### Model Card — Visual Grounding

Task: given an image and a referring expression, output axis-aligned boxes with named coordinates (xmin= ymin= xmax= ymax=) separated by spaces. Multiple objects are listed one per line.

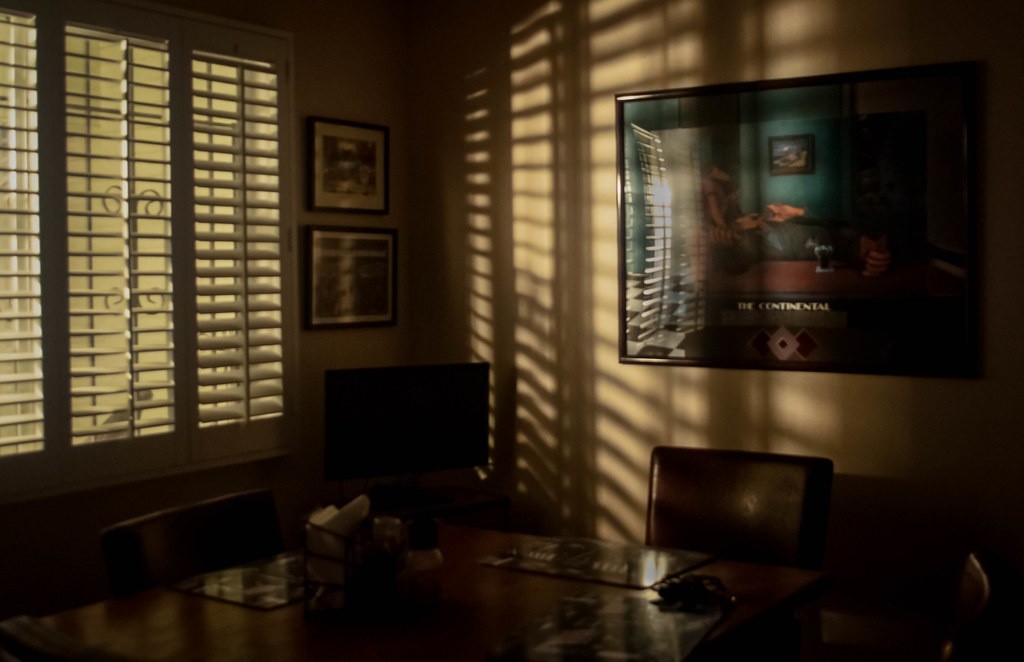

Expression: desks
xmin=0 ymin=524 xmax=836 ymax=662
xmin=372 ymin=487 xmax=514 ymax=531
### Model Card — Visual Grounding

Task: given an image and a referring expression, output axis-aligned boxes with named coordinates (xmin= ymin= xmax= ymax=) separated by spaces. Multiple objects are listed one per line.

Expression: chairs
xmin=98 ymin=486 xmax=286 ymax=596
xmin=941 ymin=544 xmax=1024 ymax=662
xmin=643 ymin=445 xmax=834 ymax=571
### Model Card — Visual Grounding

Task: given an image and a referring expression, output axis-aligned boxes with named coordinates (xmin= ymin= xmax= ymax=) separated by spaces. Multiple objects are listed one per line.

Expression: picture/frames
xmin=302 ymin=115 xmax=390 ymax=216
xmin=614 ymin=61 xmax=986 ymax=379
xmin=301 ymin=223 xmax=399 ymax=331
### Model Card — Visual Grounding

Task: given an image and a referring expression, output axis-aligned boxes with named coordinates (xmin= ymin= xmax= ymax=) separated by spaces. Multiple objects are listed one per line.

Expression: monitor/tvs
xmin=324 ymin=362 xmax=489 ymax=510
